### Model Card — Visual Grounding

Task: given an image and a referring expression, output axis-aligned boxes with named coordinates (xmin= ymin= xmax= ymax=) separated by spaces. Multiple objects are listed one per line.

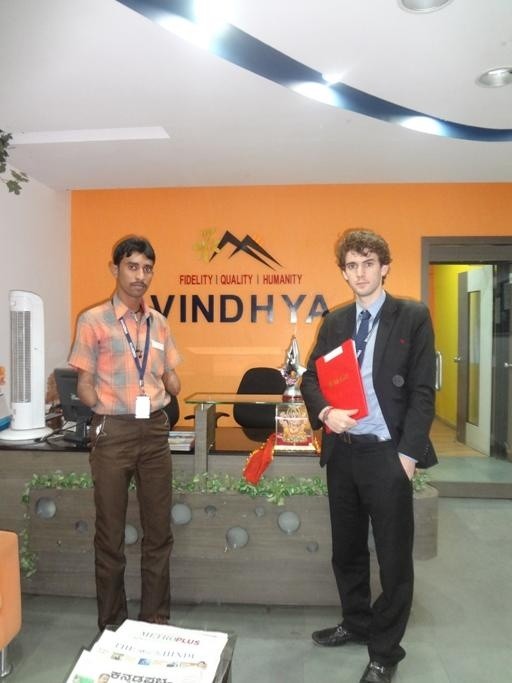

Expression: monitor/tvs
xmin=53 ymin=369 xmax=96 ymax=443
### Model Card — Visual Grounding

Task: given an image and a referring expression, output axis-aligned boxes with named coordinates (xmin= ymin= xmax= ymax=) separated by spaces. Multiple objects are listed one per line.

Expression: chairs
xmin=184 ymin=365 xmax=290 ymax=432
xmin=0 ymin=524 xmax=25 ymax=682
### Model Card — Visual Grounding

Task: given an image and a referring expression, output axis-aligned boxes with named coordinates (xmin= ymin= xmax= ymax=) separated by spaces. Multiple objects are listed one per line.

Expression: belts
xmin=109 ymin=410 xmax=162 ymax=421
xmin=336 ymin=432 xmax=387 ymax=445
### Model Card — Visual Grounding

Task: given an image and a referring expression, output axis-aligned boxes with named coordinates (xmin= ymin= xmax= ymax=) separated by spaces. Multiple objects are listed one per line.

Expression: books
xmin=314 ymin=336 xmax=369 ymax=436
xmin=67 ymin=617 xmax=230 ymax=683
xmin=167 ymin=428 xmax=196 ymax=452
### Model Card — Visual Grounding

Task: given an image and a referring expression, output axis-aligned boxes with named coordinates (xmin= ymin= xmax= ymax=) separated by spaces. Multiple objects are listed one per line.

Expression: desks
xmin=54 ymin=618 xmax=242 ymax=682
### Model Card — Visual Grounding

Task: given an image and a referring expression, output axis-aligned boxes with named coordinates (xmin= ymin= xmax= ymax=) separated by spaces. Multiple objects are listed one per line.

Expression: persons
xmin=67 ymin=232 xmax=183 ymax=632
xmin=298 ymin=225 xmax=436 ymax=682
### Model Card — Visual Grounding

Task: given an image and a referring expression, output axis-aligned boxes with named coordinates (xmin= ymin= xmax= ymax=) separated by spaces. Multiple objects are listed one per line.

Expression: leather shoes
xmin=359 ymin=659 xmax=398 ymax=682
xmin=311 ymin=623 xmax=373 ymax=648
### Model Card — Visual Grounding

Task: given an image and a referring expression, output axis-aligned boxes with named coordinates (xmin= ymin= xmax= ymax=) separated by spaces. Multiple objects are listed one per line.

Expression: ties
xmin=354 ymin=309 xmax=372 ymax=368
